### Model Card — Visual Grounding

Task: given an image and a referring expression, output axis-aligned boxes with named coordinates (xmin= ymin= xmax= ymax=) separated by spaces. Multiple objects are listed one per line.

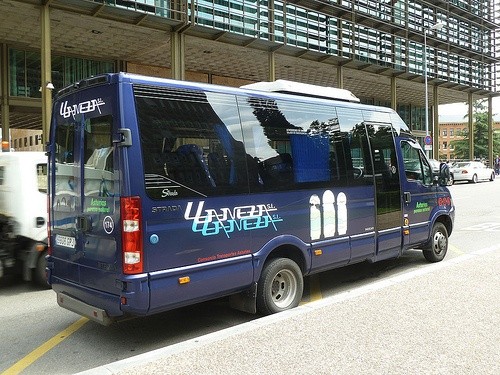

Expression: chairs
xmin=149 ymin=139 xmax=294 ymax=194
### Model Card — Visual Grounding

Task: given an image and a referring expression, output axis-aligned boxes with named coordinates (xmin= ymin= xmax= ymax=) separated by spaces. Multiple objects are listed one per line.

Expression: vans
xmin=0 ymin=142 xmax=54 ymax=289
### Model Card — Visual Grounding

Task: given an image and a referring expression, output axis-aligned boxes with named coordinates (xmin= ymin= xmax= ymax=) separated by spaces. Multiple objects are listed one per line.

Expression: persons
xmin=494 ymin=154 xmax=500 ymax=175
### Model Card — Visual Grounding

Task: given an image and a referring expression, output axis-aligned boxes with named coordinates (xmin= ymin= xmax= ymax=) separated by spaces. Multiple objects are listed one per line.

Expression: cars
xmin=450 ymin=162 xmax=496 ymax=183
xmin=385 ymin=158 xmax=454 ymax=188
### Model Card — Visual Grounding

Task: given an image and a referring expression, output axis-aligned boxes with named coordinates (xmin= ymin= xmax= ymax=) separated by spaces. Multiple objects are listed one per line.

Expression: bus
xmin=43 ymin=71 xmax=456 ymax=326
